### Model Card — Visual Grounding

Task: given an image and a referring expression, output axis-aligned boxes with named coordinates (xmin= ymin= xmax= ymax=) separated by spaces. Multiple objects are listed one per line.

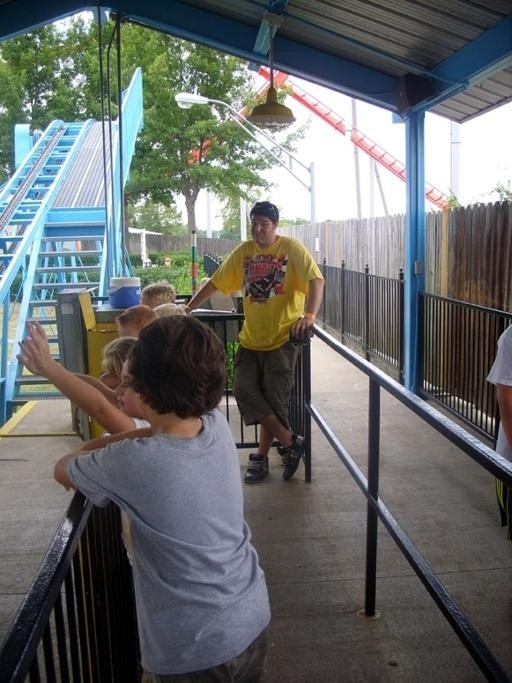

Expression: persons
xmin=52 ymin=315 xmax=275 ymax=682
xmin=183 ymin=199 xmax=325 ymax=487
xmin=483 ymin=321 xmax=512 ymax=538
xmin=28 ymin=279 xmax=180 ymax=567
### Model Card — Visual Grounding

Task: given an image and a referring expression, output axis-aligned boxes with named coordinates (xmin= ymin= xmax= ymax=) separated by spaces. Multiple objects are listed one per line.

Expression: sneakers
xmin=278 ymin=433 xmax=306 ymax=478
xmin=244 ymin=454 xmax=268 ymax=483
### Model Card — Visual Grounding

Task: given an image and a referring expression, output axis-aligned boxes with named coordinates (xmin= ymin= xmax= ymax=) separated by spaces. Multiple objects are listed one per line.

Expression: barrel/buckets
xmin=106 ymin=277 xmax=141 ymax=309
